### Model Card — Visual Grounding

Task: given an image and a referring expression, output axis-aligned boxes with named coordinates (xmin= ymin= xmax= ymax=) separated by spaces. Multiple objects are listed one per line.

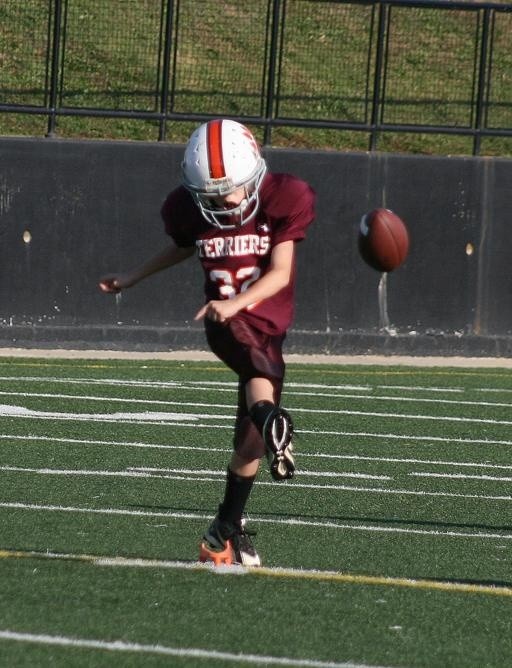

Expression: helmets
xmin=181 ymin=115 xmax=267 ymax=198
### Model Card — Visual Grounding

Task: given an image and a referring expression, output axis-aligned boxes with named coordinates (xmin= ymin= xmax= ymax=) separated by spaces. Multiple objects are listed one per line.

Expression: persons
xmin=98 ymin=116 xmax=315 ymax=569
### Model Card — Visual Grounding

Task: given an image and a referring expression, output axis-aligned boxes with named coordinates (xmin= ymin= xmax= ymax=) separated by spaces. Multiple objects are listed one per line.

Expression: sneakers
xmin=203 ymin=512 xmax=262 ymax=566
xmin=260 ymin=405 xmax=297 ymax=481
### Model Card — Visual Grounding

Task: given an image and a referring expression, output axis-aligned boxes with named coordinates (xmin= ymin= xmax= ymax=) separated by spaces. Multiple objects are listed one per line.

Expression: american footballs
xmin=358 ymin=209 xmax=410 ymax=272
